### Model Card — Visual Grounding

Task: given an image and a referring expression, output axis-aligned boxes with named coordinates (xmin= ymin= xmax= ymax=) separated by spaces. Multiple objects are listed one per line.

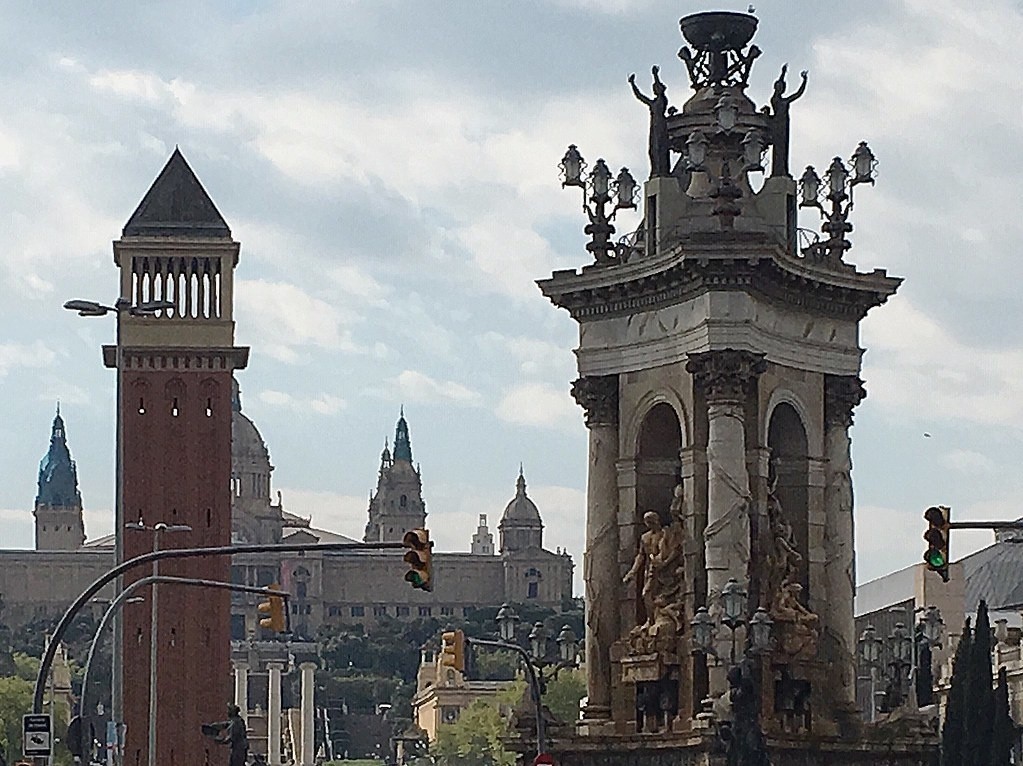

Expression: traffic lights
xmin=921 ymin=505 xmax=951 ymax=575
xmin=443 ymin=628 xmax=464 ymax=671
xmin=257 ymin=585 xmax=287 ymax=633
xmin=402 ymin=529 xmax=434 ymax=593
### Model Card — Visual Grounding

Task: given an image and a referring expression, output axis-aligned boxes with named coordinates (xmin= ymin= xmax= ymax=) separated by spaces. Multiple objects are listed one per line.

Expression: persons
xmin=768 ymin=458 xmax=818 ymax=656
xmin=622 ymin=482 xmax=686 ymax=631
xmin=724 ymin=666 xmax=771 ymax=766
xmin=201 ymin=702 xmax=250 ymax=766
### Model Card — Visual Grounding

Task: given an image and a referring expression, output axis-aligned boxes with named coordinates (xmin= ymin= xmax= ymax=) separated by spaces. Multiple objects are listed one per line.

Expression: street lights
xmin=124 ymin=521 xmax=192 ymax=766
xmin=62 ymin=295 xmax=181 ymax=766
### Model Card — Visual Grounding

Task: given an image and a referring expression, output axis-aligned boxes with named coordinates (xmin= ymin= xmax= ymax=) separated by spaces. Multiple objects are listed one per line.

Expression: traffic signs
xmin=22 ymin=713 xmax=54 ymax=759
